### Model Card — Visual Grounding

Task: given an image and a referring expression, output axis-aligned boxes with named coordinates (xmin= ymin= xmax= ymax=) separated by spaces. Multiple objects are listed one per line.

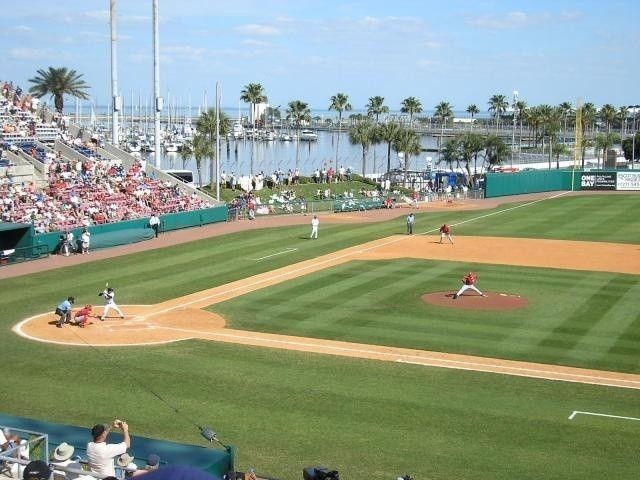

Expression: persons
xmin=50 ymin=230 xmax=78 ymax=256
xmin=439 ymin=224 xmax=455 ymax=246
xmin=0 ymin=427 xmax=161 ymax=480
xmin=150 ymin=213 xmax=161 ymax=239
xmin=406 ymin=213 xmax=415 ymax=236
xmin=84 ymin=419 xmax=132 ymax=479
xmin=0 ymin=78 xmax=214 ymax=236
xmin=309 ymin=214 xmax=320 ymax=240
xmin=217 ymin=165 xmax=470 ymax=221
xmin=72 ymin=302 xmax=94 ymax=329
xmin=79 ymin=227 xmax=91 ymax=255
xmin=54 ymin=296 xmax=76 ymax=328
xmin=97 ymin=286 xmax=127 ymax=322
xmin=453 ymin=271 xmax=488 ymax=300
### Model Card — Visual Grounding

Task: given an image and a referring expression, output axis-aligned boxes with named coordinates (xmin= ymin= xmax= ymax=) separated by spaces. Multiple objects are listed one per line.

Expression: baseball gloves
xmin=462 ymin=280 xmax=465 ymax=283
xmin=93 ymin=314 xmax=98 ymax=318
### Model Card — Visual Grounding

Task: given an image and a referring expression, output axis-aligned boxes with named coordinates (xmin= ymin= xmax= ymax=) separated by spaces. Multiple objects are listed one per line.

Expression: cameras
xmin=117 ymin=422 xmax=123 ymax=426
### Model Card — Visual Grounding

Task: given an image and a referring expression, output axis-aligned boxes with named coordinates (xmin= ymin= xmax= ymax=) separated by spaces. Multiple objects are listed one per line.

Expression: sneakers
xmin=58 ymin=323 xmax=62 ymax=328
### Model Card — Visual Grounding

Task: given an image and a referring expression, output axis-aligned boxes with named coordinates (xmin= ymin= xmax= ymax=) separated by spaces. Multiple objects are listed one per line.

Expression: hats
xmin=146 ymin=454 xmax=160 ymax=466
xmin=23 ymin=460 xmax=53 ymax=479
xmin=54 ymin=442 xmax=74 ymax=461
xmin=92 ymin=423 xmax=108 ymax=436
xmin=69 ymin=297 xmax=74 ymax=304
xmin=118 ymin=453 xmax=134 ymax=466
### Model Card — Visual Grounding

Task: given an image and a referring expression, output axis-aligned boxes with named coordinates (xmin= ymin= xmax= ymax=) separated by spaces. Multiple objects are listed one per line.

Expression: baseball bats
xmin=105 ymin=282 xmax=108 ymax=294
